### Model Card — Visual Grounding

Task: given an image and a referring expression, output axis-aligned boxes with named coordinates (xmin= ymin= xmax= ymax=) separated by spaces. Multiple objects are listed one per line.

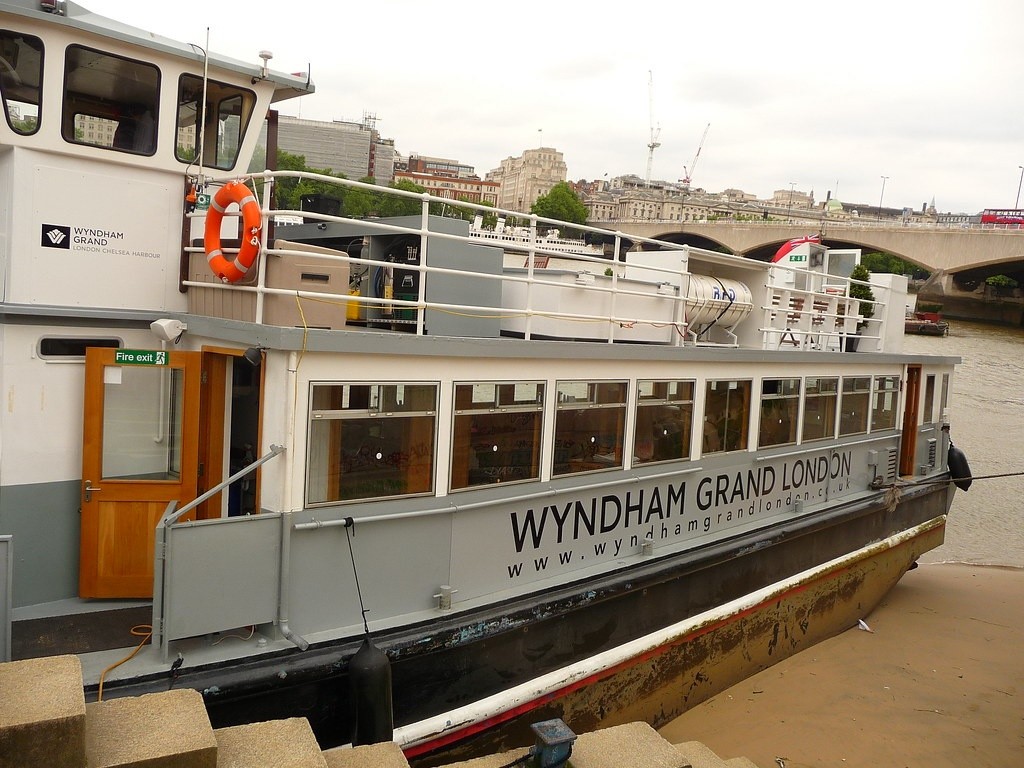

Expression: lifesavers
xmin=204 ymin=183 xmax=261 ymax=282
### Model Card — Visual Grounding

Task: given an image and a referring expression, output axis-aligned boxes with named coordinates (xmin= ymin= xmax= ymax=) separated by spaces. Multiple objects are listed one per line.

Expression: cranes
xmin=678 ymin=123 xmax=711 ymax=183
xmin=645 ymin=68 xmax=661 ymax=189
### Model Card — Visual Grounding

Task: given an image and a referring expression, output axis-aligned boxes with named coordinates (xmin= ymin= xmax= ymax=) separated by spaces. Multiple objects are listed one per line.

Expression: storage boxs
xmin=189 ymin=238 xmax=349 ymax=331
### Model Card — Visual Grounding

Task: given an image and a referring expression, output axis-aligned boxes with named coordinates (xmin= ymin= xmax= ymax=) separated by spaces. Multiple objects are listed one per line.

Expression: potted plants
xmin=914 ymin=301 xmax=943 ymax=322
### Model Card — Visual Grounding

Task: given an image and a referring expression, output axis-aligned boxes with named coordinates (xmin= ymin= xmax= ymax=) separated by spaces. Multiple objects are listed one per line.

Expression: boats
xmin=2 ymin=0 xmax=974 ymax=768
xmin=905 ymin=311 xmax=949 ymax=338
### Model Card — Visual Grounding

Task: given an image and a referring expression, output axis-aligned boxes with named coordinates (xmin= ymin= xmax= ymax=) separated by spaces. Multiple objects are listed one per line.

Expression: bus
xmin=979 ymin=208 xmax=1024 ymax=225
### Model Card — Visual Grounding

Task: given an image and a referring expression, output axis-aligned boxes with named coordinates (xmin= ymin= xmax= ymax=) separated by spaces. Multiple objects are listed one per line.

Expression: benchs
xmin=771 ymin=296 xmax=850 ymax=351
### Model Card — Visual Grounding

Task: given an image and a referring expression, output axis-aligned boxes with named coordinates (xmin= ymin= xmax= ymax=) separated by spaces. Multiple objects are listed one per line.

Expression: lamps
xmin=243 ymin=345 xmax=268 ymax=366
xmin=259 ymin=50 xmax=272 ymax=78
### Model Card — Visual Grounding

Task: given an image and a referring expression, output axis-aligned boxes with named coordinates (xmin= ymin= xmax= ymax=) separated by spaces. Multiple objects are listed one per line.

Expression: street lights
xmin=877 ymin=176 xmax=889 ymax=222
xmin=1015 ymin=165 xmax=1024 ymax=210
xmin=787 ymin=182 xmax=797 ymax=220
